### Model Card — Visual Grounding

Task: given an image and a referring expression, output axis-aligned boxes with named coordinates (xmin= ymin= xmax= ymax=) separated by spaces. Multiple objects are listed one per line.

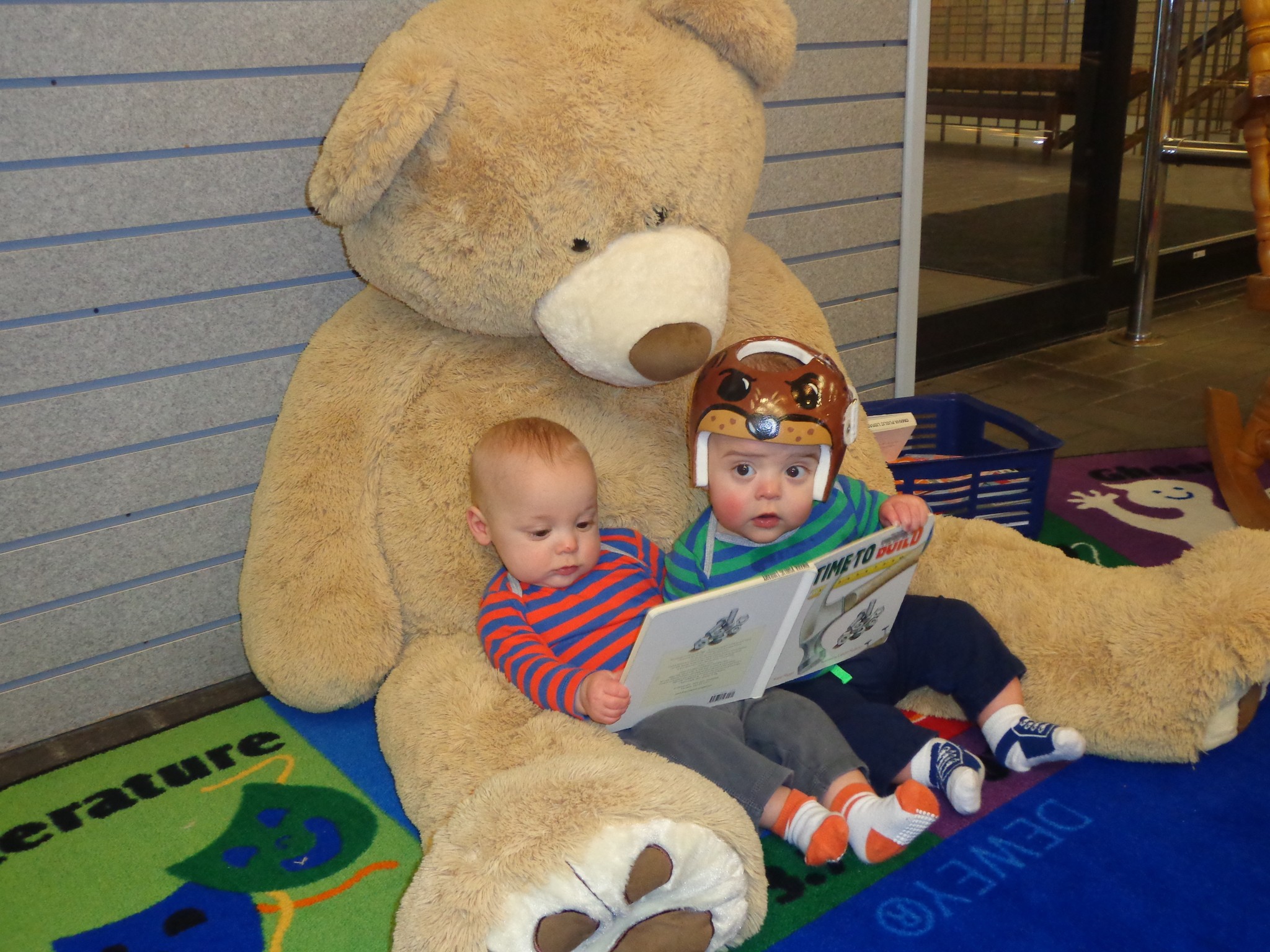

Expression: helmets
xmin=686 ymin=335 xmax=858 ymax=503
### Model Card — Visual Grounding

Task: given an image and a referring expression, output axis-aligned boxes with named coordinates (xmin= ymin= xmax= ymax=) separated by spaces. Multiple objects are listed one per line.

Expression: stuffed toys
xmin=239 ymin=0 xmax=1270 ymax=952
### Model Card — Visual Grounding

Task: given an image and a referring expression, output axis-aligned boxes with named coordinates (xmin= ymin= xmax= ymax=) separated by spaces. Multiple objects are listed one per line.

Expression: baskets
xmin=863 ymin=389 xmax=1063 ymax=537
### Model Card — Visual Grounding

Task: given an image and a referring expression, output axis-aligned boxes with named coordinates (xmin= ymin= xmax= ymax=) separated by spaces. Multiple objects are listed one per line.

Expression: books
xmin=594 ymin=504 xmax=931 ymax=736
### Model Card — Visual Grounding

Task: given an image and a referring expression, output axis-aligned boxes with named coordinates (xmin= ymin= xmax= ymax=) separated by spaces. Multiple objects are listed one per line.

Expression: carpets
xmin=1 ymin=443 xmax=1270 ymax=952
xmin=918 ymin=191 xmax=1256 ymax=288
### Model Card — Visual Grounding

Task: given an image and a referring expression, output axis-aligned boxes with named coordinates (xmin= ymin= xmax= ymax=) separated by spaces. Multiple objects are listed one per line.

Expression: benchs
xmin=928 ymin=63 xmax=1145 ymax=162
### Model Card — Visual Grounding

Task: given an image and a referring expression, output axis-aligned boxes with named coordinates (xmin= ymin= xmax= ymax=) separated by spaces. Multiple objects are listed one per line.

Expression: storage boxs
xmin=862 ymin=393 xmax=1067 ymax=538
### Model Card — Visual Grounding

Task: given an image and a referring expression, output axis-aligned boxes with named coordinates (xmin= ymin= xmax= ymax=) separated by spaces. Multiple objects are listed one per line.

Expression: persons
xmin=464 ymin=419 xmax=940 ymax=867
xmin=664 ymin=336 xmax=1083 ymax=817
